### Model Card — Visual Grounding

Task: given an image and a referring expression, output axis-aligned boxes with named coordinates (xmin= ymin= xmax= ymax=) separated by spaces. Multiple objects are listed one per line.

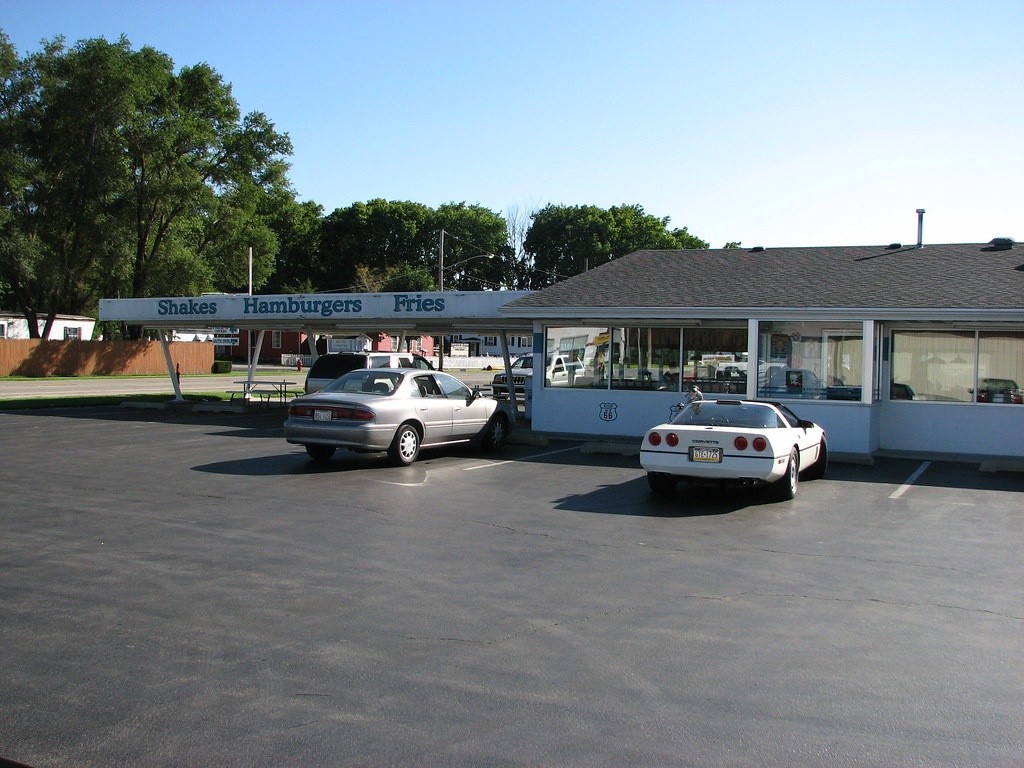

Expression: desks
xmin=234 ymin=381 xmax=297 ymax=409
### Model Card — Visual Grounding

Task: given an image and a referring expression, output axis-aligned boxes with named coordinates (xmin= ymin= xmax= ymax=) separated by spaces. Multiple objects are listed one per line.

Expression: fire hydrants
xmin=297 ymin=358 xmax=303 ymax=371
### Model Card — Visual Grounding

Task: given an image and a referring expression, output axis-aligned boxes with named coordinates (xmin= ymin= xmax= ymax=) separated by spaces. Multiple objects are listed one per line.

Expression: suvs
xmin=304 ymin=350 xmax=438 ymax=396
xmin=492 ymin=352 xmax=585 ymax=397
xmin=759 ymin=366 xmax=826 ymax=399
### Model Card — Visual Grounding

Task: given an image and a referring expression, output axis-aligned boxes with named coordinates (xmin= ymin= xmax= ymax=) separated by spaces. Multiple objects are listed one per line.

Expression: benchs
xmin=373 ymin=383 xmax=427 ymax=398
xmin=227 ymin=389 xmax=304 ymax=407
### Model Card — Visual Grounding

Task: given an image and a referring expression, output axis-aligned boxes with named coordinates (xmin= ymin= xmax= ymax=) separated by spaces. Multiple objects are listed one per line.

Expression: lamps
xmin=580 ymin=319 xmax=702 ymax=326
xmin=951 ymin=322 xmax=1024 ymax=330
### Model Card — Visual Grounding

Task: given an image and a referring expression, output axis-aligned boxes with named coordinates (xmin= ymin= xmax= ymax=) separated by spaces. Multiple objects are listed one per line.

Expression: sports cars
xmin=639 ymin=399 xmax=829 ymax=500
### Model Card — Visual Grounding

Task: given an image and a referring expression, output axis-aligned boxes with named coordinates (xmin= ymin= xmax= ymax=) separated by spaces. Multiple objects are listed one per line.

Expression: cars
xmin=283 ymin=368 xmax=515 ymax=467
xmin=819 ymin=382 xmax=917 ymax=401
xmin=968 ymin=378 xmax=1021 ymax=404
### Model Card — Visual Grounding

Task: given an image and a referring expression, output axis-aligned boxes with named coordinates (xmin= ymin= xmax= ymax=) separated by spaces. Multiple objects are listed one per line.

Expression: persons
xmin=664 ymin=362 xmax=676 ymax=383
xmin=594 ymin=351 xmax=605 ymax=379
